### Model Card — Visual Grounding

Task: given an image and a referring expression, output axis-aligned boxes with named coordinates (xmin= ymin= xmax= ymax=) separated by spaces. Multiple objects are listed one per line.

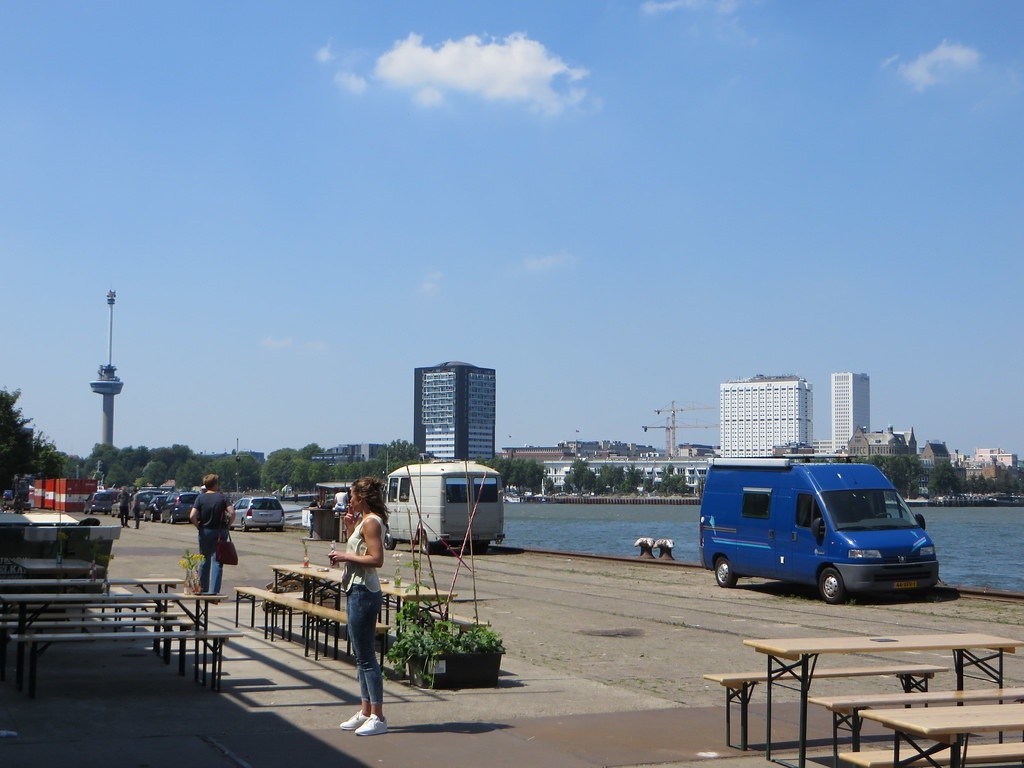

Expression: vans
xmin=699 ymin=457 xmax=939 ymax=604
xmin=384 ymin=461 xmax=507 ymax=556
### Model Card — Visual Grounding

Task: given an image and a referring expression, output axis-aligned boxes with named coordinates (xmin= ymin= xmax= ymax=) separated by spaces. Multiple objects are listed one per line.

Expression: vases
xmin=395 ymin=568 xmax=401 ymax=588
xmin=185 ymin=571 xmax=202 ymax=594
xmin=101 ymin=578 xmax=111 ymax=597
xmin=55 ymin=552 xmax=63 ymax=564
xmin=89 ymin=563 xmax=97 ymax=581
xmin=303 ymin=552 xmax=309 ymax=569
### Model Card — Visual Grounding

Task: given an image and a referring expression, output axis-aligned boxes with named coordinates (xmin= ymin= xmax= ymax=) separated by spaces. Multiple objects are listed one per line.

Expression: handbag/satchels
xmin=216 ymin=542 xmax=238 ymax=565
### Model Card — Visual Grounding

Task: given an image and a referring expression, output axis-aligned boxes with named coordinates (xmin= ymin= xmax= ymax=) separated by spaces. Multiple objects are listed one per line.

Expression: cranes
xmin=642 ymin=416 xmax=720 ymax=459
xmin=654 ymin=400 xmax=717 ymax=457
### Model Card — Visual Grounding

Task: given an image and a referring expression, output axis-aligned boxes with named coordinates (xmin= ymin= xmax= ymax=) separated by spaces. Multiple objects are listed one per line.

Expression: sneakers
xmin=340 ymin=710 xmax=370 ymax=730
xmin=355 ymin=714 xmax=387 ymax=735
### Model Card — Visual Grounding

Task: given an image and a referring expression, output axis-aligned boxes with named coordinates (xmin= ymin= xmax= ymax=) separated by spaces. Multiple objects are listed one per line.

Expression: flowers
xmin=56 ymin=529 xmax=70 ymax=555
xmin=89 ymin=542 xmax=101 ymax=561
xmin=303 ymin=540 xmax=310 ymax=556
xmin=102 ymin=555 xmax=114 ymax=578
xmin=185 ymin=552 xmax=205 ymax=574
xmin=391 ymin=552 xmax=406 ymax=564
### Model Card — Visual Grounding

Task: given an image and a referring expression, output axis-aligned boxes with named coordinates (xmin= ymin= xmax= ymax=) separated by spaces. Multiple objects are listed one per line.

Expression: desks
xmin=0 ymin=577 xmax=189 ymax=666
xmin=858 ymin=703 xmax=1024 ymax=768
xmin=11 ymin=558 xmax=106 ymax=604
xmin=272 ymin=563 xmax=457 ymax=662
xmin=1 ymin=593 xmax=227 ymax=694
xmin=742 ymin=630 xmax=1024 ymax=768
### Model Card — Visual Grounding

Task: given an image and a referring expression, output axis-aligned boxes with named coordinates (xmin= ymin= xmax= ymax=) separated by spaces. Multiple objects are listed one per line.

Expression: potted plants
xmin=386 ymin=559 xmax=504 ymax=694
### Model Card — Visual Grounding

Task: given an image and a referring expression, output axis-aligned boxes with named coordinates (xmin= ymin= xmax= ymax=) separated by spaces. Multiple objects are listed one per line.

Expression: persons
xmin=117 ymin=486 xmax=130 ymax=528
xmin=132 ymin=488 xmax=140 ymax=529
xmin=334 ymin=488 xmax=348 ymax=504
xmin=309 ymin=496 xmax=319 ymax=538
xmin=190 ymin=474 xmax=235 ymax=602
xmin=328 ymin=478 xmax=388 ymax=735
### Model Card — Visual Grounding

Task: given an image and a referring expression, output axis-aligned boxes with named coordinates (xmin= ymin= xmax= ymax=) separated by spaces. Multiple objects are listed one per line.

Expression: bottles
xmin=329 ymin=540 xmax=340 ymax=568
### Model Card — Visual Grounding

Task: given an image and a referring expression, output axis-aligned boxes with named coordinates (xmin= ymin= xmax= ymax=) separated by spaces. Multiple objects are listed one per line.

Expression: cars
xmin=110 ymin=489 xmax=167 ymax=523
xmin=83 ymin=492 xmax=114 ymax=515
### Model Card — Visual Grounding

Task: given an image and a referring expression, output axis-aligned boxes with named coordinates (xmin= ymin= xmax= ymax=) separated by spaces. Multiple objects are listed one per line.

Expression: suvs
xmin=228 ymin=495 xmax=286 ymax=532
xmin=160 ymin=490 xmax=200 ymax=524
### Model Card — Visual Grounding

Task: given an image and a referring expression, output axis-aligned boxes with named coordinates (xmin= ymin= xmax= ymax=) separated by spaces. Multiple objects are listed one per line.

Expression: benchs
xmin=0 ymin=604 xmax=242 ymax=698
xmin=234 ymin=583 xmax=494 ymax=674
xmin=704 ymin=663 xmax=1024 ymax=768
